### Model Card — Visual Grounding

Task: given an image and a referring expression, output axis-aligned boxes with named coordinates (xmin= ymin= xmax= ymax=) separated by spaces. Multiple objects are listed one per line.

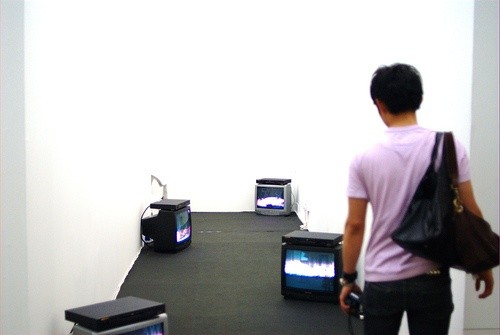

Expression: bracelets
xmin=339 ymin=271 xmax=358 ymax=286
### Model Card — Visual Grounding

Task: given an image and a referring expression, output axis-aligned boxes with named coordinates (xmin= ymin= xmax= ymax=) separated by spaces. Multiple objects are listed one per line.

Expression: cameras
xmin=347 ymin=292 xmax=365 ymax=319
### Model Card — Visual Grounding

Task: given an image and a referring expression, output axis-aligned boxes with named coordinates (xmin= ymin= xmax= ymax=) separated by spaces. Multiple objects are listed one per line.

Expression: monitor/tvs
xmin=281 ymin=244 xmax=342 ymax=305
xmin=255 ymin=183 xmax=291 ymax=215
xmin=74 ymin=313 xmax=169 ymax=335
xmin=142 ymin=206 xmax=192 ymax=254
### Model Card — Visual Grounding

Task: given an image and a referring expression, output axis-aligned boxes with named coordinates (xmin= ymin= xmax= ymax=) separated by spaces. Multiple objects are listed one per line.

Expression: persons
xmin=338 ymin=62 xmax=494 ymax=334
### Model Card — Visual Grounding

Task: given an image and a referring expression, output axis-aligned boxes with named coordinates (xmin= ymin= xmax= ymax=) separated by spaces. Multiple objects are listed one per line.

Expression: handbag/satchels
xmin=390 ymin=132 xmax=500 ymax=274
